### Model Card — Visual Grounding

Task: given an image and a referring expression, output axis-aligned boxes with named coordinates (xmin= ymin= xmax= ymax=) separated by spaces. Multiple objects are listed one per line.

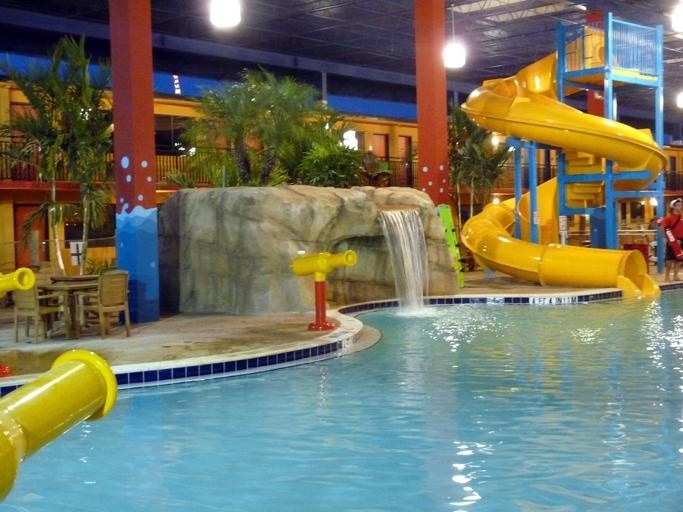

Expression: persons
xmin=657 ymin=198 xmax=683 ymax=282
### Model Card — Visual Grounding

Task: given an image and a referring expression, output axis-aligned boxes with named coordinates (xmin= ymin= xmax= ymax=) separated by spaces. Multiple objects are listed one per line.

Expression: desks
xmin=40 ymin=274 xmax=102 ymax=339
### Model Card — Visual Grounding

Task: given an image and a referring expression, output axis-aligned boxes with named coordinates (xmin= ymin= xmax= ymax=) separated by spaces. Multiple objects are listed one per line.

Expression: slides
xmin=460 ymin=26 xmax=669 ymax=302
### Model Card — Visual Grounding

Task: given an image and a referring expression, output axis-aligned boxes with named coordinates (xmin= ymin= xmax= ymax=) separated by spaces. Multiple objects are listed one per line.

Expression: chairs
xmin=12 ymin=286 xmax=71 ymax=343
xmin=73 ymin=272 xmax=131 ymax=341
